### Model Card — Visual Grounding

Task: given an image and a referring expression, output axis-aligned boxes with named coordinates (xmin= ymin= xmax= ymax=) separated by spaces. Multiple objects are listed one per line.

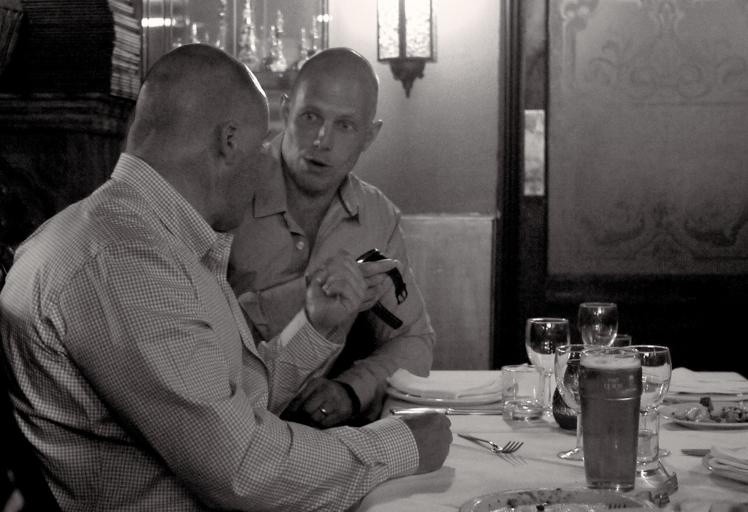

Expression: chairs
xmin=0 ymin=241 xmax=60 ymax=511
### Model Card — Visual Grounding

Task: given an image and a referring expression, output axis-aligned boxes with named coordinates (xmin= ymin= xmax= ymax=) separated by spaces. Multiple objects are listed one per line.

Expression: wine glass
xmin=552 ymin=343 xmax=589 ymax=463
xmin=578 ymin=299 xmax=620 ymax=347
xmin=524 ymin=315 xmax=573 ymax=424
xmin=627 ymin=342 xmax=676 ymax=489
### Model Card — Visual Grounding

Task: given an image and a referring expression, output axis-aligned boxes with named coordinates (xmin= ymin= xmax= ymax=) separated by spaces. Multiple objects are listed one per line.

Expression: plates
xmin=656 ymin=395 xmax=748 ymax=430
xmin=456 ymin=481 xmax=664 ymax=512
xmin=384 ymin=384 xmax=512 ymax=406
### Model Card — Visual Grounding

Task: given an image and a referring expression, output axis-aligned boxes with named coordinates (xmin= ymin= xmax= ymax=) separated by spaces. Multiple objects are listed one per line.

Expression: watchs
xmin=354 ymin=248 xmax=411 ymax=331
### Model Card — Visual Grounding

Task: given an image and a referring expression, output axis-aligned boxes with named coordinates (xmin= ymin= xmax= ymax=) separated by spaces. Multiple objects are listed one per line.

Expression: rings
xmin=318 ymin=406 xmax=330 ymax=417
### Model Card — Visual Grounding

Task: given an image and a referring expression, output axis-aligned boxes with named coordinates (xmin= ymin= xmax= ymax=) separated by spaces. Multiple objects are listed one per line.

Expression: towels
xmin=707 ymin=438 xmax=747 ymax=483
xmin=385 ymin=367 xmax=504 ymax=402
xmin=668 ymin=364 xmax=747 ymax=398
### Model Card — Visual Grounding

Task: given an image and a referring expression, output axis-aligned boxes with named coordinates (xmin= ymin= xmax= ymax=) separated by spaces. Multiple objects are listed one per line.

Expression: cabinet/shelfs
xmin=1 ymin=93 xmax=138 ymax=248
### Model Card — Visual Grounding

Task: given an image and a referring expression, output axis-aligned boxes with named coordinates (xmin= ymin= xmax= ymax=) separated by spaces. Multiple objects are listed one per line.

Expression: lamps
xmin=374 ymin=1 xmax=437 ymax=99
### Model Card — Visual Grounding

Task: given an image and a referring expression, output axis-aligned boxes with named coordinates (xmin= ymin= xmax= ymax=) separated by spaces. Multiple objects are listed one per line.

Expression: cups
xmin=499 ymin=365 xmax=548 ymax=424
xmin=577 ymin=346 xmax=645 ymax=494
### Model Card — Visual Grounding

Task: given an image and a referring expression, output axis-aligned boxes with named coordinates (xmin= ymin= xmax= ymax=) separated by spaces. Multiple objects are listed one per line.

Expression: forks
xmin=456 ymin=431 xmax=526 ymax=454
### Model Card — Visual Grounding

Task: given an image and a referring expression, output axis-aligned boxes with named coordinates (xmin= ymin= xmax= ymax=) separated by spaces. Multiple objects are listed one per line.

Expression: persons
xmin=226 ymin=45 xmax=441 ymax=431
xmin=0 ymin=42 xmax=453 ymax=511
